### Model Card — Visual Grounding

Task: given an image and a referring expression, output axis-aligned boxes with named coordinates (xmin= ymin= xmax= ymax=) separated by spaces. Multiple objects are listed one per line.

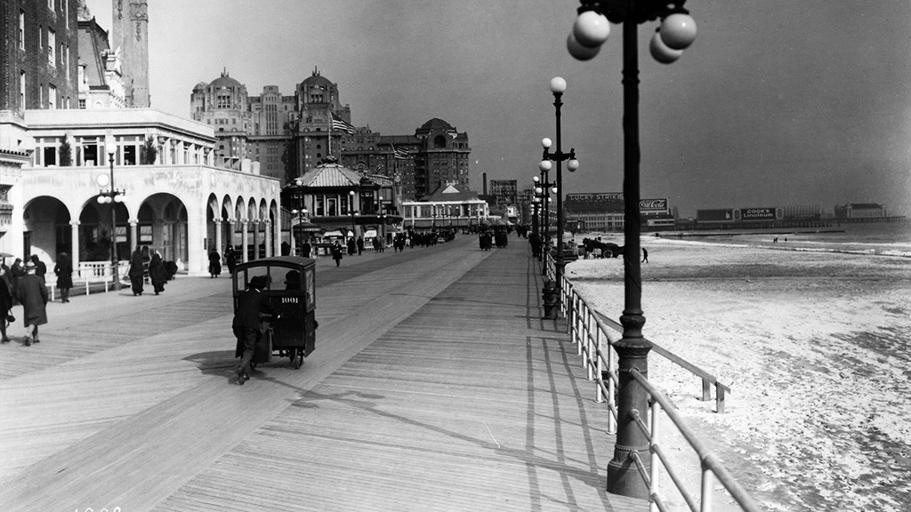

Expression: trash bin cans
xmin=541 ymin=279 xmax=563 ymax=321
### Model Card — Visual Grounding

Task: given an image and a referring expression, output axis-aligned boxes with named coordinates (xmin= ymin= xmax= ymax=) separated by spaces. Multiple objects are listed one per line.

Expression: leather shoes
xmin=235 ymin=365 xmax=249 ymax=386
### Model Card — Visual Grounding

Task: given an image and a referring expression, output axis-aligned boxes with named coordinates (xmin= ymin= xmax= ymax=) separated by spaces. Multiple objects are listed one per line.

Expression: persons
xmin=346 ymin=236 xmax=364 ymax=256
xmin=128 ymin=245 xmax=178 ymax=296
xmin=209 ymin=248 xmax=221 ymax=278
xmin=372 ymin=236 xmax=385 ymax=253
xmin=232 ymin=275 xmax=281 ymax=386
xmin=303 ymin=239 xmax=311 ymax=258
xmin=1 ymin=255 xmax=48 ymax=346
xmin=393 ymin=228 xmax=437 ymax=253
xmin=284 ymin=269 xmax=310 ymax=300
xmin=641 ymin=247 xmax=650 ymax=263
xmin=331 ymin=240 xmax=343 ymax=267
xmin=224 ymin=245 xmax=237 ymax=278
xmin=441 ymin=228 xmax=455 ymax=242
xmin=462 ymin=224 xmax=530 ymax=239
xmin=54 ymin=252 xmax=73 ymax=303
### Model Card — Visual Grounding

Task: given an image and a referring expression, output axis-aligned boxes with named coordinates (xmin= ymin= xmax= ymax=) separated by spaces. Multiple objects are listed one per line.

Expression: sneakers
xmin=22 ymin=336 xmax=32 ymax=347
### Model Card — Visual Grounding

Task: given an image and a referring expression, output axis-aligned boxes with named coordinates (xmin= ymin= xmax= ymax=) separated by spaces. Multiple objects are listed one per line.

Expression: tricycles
xmin=232 ymin=255 xmax=316 ymax=367
xmin=478 ymin=231 xmax=507 ymax=251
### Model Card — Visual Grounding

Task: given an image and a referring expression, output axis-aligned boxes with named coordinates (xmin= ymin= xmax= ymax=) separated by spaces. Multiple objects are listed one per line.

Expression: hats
xmin=21 ymin=261 xmax=38 ymax=271
xmin=282 ymin=270 xmax=301 ymax=285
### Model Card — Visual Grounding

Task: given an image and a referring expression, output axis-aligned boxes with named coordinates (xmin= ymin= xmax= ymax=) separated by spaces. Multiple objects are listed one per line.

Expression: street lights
xmin=346 ymin=189 xmax=359 ymax=246
xmin=525 ymin=136 xmax=579 ymax=278
xmin=557 ymin=0 xmax=697 ymax=496
xmin=97 ymin=142 xmax=126 ymax=291
xmin=376 ymin=196 xmax=387 ymax=239
xmin=538 ymin=76 xmax=580 ymax=322
xmin=291 ymin=177 xmax=308 ymax=255
